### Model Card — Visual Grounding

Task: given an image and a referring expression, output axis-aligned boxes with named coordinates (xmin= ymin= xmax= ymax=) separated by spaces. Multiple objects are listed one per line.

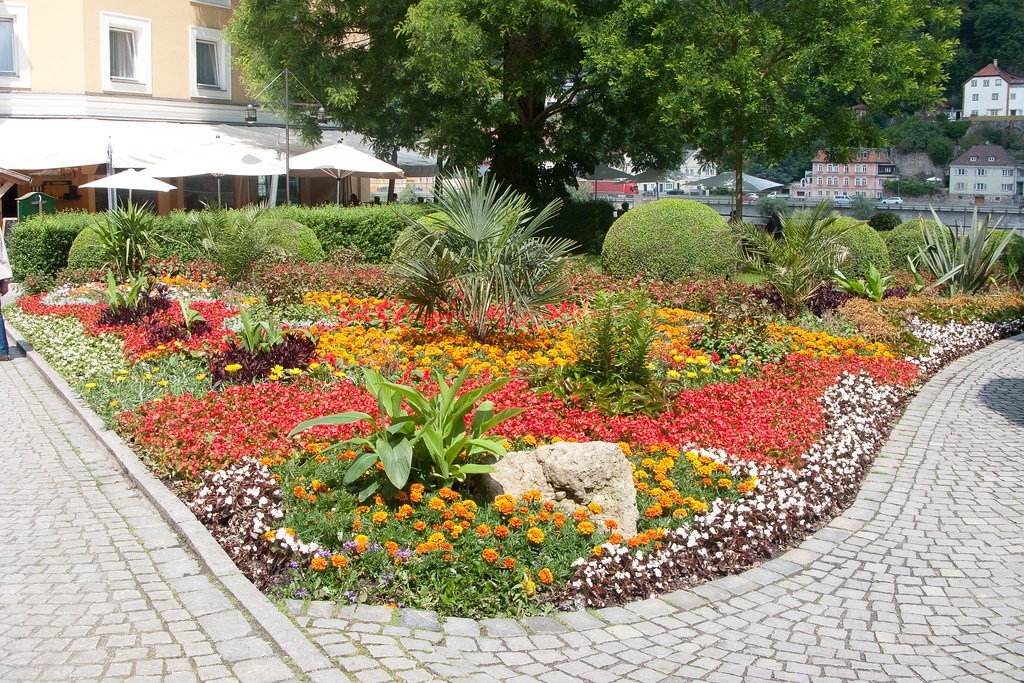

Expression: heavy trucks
xmin=589 ymin=181 xmax=639 ymax=198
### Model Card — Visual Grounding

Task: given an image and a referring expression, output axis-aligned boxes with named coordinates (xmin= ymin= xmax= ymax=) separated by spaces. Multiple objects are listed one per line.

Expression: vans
xmin=834 ymin=195 xmax=854 ymax=204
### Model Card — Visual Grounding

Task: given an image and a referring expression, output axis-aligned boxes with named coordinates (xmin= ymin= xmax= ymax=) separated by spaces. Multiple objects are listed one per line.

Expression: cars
xmin=667 ymin=189 xmax=684 ymax=195
xmin=742 ymin=193 xmax=760 ymax=203
xmin=373 ymin=187 xmax=389 ymax=196
xmin=882 ymin=197 xmax=903 ymax=204
xmin=642 ymin=191 xmax=656 ymax=197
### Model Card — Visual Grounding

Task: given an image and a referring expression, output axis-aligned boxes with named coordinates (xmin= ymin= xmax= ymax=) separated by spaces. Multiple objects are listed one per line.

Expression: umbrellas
xmin=150 ymin=136 xmax=287 ymax=205
xmin=289 ymin=140 xmax=405 ymax=205
xmin=682 ymin=171 xmax=784 ymax=222
xmin=572 ymin=165 xmax=631 ymax=200
xmin=616 ymin=167 xmax=702 ymax=200
xmin=78 ymin=168 xmax=177 ymax=200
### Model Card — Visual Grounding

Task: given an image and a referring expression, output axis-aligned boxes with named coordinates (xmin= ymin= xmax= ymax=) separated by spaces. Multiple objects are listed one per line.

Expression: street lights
xmin=244 ymin=68 xmax=328 ymax=202
xmin=896 ymin=177 xmax=899 ymax=197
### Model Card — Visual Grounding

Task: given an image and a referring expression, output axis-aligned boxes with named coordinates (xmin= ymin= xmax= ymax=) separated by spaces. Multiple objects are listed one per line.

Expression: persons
xmin=653 ymin=187 xmax=656 ymax=194
xmin=727 ymin=210 xmax=737 ymax=223
xmin=394 ymin=193 xmax=397 ymax=202
xmin=374 ymin=196 xmax=381 ymax=205
xmin=764 ymin=210 xmax=784 ymax=239
xmin=0 ymin=230 xmax=13 ymax=360
xmin=348 ymin=194 xmax=361 ymax=205
xmin=621 ymin=202 xmax=629 ymax=211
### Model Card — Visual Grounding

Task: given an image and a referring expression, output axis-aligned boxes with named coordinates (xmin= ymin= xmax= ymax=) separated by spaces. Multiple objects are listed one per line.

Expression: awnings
xmin=229 ymin=123 xmax=486 ymax=178
xmin=108 ymin=122 xmax=289 ymax=168
xmin=0 ymin=119 xmax=109 ymax=170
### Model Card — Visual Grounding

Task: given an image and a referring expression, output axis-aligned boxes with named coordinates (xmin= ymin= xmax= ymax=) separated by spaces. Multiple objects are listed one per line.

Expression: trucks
xmin=767 ymin=191 xmax=790 ymax=200
